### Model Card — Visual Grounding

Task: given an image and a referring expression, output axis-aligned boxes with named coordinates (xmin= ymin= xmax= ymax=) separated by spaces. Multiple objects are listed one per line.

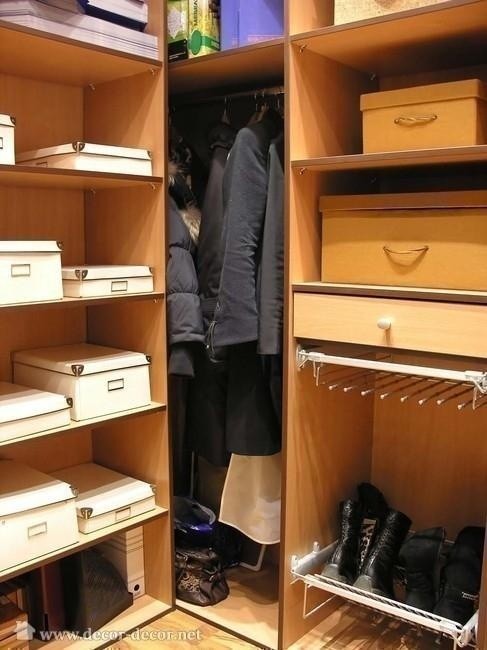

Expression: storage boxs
xmin=0 ymin=457 xmax=78 ymax=573
xmin=317 ymin=189 xmax=487 ymax=291
xmin=0 ymin=237 xmax=64 ymax=304
xmin=47 ymin=461 xmax=156 ymax=537
xmin=361 ymin=78 xmax=487 ymax=154
xmin=10 ymin=339 xmax=153 ymax=423
xmin=13 ymin=140 xmax=152 ymax=177
xmin=60 ymin=263 xmax=152 ymax=299
xmin=1 ymin=380 xmax=72 ymax=446
xmin=0 ymin=114 xmax=17 ymax=165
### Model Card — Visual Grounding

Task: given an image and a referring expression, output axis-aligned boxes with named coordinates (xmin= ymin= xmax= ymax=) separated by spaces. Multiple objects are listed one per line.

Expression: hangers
xmin=215 ymin=85 xmax=284 ymax=127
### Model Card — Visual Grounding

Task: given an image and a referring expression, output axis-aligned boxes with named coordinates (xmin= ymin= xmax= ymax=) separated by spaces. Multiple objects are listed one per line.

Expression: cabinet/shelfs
xmin=0 ymin=1 xmax=179 ymax=650
xmin=166 ymin=1 xmax=487 ymax=650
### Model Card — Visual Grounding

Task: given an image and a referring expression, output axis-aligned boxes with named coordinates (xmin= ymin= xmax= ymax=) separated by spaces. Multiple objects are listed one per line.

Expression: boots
xmin=321 ymin=483 xmax=484 ymax=629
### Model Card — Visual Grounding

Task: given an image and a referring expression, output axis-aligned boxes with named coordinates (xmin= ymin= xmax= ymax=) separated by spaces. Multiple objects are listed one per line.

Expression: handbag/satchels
xmin=176 ymin=547 xmax=229 ymax=606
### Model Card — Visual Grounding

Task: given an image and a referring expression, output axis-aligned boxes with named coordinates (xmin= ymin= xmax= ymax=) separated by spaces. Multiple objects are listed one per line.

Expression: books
xmin=166 ymin=1 xmax=283 ymax=61
